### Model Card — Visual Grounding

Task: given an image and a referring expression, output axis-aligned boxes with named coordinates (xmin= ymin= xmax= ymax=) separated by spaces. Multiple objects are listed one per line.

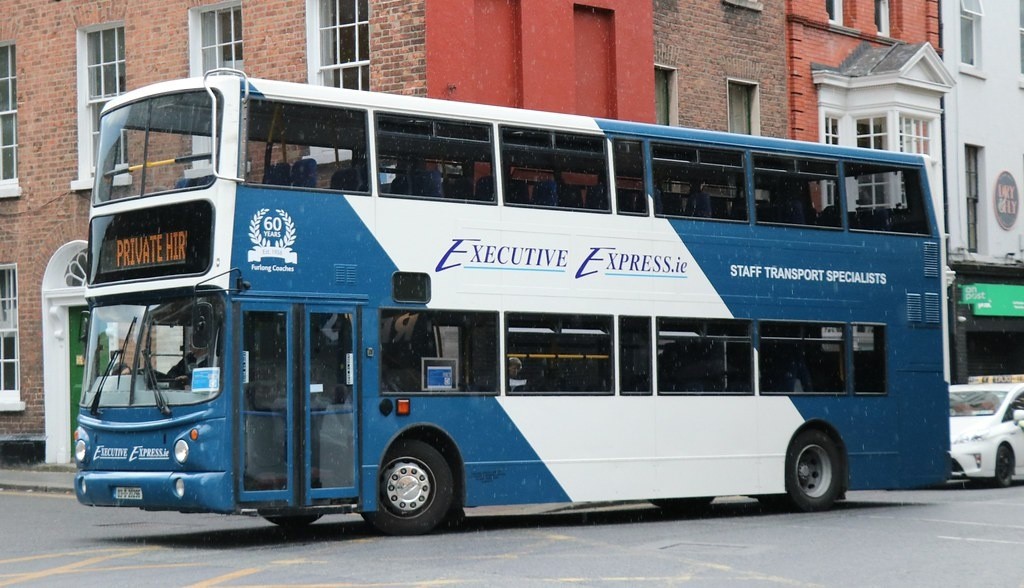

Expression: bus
xmin=72 ymin=67 xmax=953 ymax=537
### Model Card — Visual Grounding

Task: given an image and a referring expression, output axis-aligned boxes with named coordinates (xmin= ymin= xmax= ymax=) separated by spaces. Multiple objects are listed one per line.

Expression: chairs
xmin=175 ymin=158 xmax=896 ymax=391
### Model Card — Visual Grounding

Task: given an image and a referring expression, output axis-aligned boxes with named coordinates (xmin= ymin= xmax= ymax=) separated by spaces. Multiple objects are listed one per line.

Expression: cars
xmin=949 ymin=374 xmax=1024 ymax=491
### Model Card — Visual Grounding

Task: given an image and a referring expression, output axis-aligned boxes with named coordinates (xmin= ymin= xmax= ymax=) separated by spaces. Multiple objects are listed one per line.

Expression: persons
xmin=174 ymin=334 xmax=211 ymax=391
xmin=113 ymin=363 xmax=132 ymax=376
